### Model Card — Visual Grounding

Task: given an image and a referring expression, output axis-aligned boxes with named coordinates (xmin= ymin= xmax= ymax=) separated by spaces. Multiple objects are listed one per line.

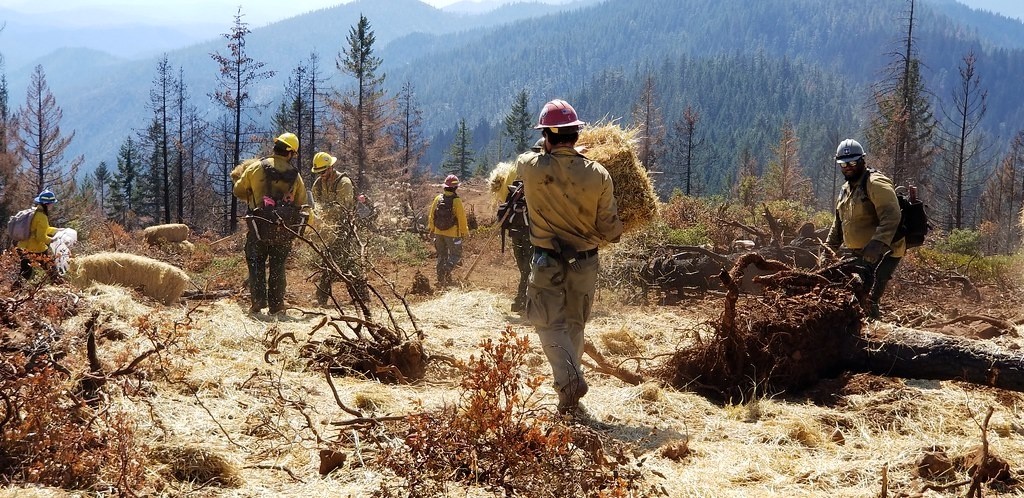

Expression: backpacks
xmin=7 ymin=207 xmax=44 ymax=241
xmin=861 ymin=166 xmax=927 ymax=248
xmin=497 ymin=179 xmax=529 ymax=229
xmin=316 ymin=173 xmax=374 ymax=219
xmin=433 ymin=194 xmax=459 ymax=230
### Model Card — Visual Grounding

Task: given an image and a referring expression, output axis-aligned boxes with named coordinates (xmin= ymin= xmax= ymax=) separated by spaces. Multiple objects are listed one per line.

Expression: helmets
xmin=274 ymin=133 xmax=299 ymax=157
xmin=311 ymin=152 xmax=337 ymax=173
xmin=34 ymin=189 xmax=57 ymax=204
xmin=534 ymin=99 xmax=584 ymax=129
xmin=442 ymin=175 xmax=461 ymax=188
xmin=835 ymin=139 xmax=866 ymax=163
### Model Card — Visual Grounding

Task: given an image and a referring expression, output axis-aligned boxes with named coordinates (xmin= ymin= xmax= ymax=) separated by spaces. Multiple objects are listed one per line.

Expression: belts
xmin=534 ymin=245 xmax=598 ymax=261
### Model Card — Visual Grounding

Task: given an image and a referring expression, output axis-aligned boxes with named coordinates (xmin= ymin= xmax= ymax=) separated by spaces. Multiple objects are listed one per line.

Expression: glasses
xmin=318 ymin=169 xmax=327 ymax=174
xmin=839 ymin=157 xmax=861 ymax=168
xmin=542 ymin=129 xmax=546 ymax=138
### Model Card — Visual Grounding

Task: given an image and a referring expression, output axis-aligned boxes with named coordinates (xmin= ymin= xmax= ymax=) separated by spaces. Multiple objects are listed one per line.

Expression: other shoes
xmin=314 ymin=296 xmax=327 ymax=308
xmin=438 ymin=273 xmax=452 ymax=284
xmin=348 ymin=297 xmax=371 ymax=307
xmin=250 ymin=298 xmax=267 ymax=312
xmin=267 ymin=302 xmax=285 ymax=315
xmin=557 ymin=377 xmax=587 ymax=414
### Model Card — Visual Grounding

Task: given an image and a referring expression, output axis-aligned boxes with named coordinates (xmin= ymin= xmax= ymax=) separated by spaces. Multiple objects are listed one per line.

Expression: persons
xmin=517 ymin=98 xmax=623 ymax=411
xmin=310 ymin=152 xmax=370 ymax=308
xmin=825 ymin=138 xmax=905 ymax=321
xmin=12 ymin=190 xmax=62 ymax=292
xmin=504 ymin=179 xmax=534 ymax=312
xmin=232 ymin=133 xmax=309 ymax=316
xmin=429 ymin=174 xmax=470 ymax=283
